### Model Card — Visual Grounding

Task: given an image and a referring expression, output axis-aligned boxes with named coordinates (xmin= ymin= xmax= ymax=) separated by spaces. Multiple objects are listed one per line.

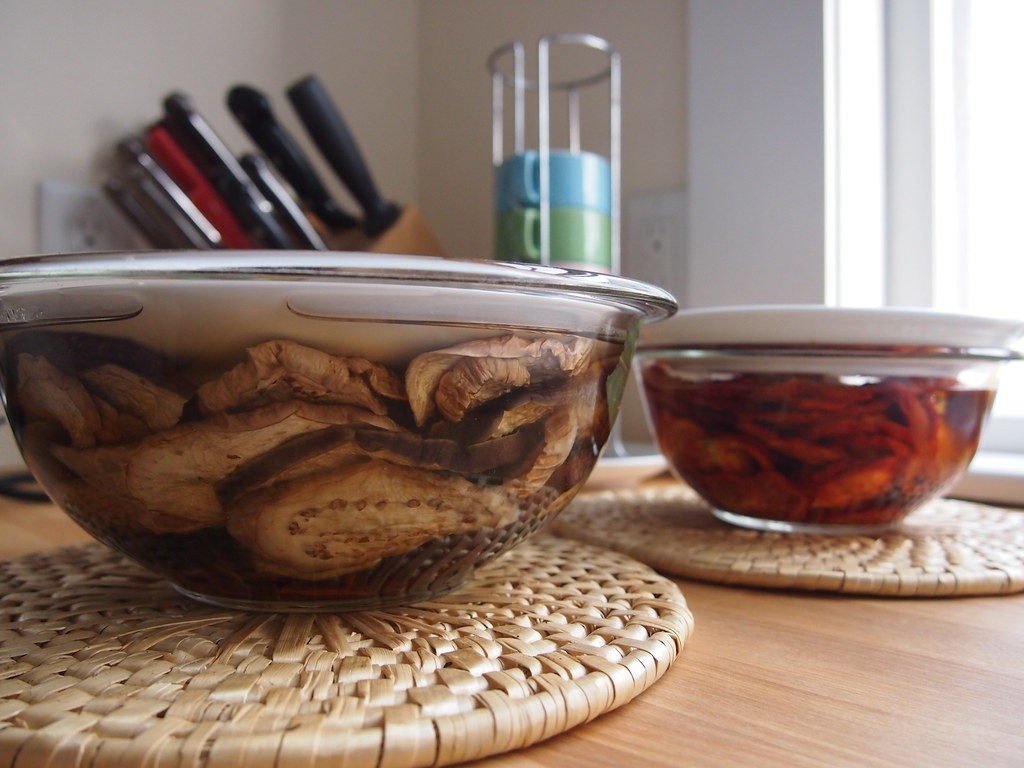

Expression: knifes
xmin=101 ymin=73 xmax=396 ymax=252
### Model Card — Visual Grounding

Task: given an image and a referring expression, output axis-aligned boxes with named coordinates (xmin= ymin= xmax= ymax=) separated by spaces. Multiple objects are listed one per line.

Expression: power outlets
xmin=35 ymin=183 xmax=135 ymax=257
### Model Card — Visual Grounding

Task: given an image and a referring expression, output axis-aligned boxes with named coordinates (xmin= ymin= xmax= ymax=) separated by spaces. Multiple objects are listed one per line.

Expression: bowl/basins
xmin=632 ymin=297 xmax=1023 ymax=539
xmin=1 ymin=244 xmax=680 ymax=616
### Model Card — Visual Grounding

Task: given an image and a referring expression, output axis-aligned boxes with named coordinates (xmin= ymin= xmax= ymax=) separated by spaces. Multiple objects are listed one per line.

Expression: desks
xmin=0 ymin=451 xmax=1024 ymax=768
